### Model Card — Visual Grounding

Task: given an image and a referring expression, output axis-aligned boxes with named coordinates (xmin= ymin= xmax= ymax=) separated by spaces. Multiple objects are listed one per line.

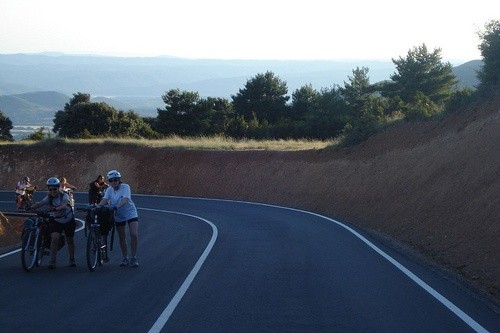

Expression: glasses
xmin=49 ymin=188 xmax=57 ymax=191
xmin=109 ymin=179 xmax=119 ymax=182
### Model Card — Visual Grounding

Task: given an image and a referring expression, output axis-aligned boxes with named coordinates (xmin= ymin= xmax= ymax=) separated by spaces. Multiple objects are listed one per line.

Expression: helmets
xmin=46 ymin=177 xmax=60 ymax=187
xmin=24 ymin=176 xmax=30 ymax=182
xmin=106 ymin=170 xmax=122 ymax=180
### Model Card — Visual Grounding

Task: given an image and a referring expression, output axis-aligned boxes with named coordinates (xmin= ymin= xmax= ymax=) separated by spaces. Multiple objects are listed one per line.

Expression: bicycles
xmin=91 ymin=185 xmax=110 ymax=207
xmin=74 ymin=206 xmax=110 ymax=272
xmin=19 ymin=185 xmax=37 ymax=211
xmin=20 ymin=207 xmax=57 ymax=271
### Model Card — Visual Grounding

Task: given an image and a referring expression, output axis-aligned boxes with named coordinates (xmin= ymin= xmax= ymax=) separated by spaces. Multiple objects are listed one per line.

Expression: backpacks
xmin=86 ymin=207 xmax=114 ymax=231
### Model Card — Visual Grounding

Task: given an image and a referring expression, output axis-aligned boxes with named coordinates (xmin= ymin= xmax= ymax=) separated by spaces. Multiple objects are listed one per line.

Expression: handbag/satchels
xmin=21 ymin=217 xmax=65 ymax=251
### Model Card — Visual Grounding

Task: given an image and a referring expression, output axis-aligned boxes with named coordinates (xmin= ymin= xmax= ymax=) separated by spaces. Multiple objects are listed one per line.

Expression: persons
xmin=15 ymin=176 xmax=32 ymax=209
xmin=88 ymin=174 xmax=110 ymax=206
xmin=59 ymin=177 xmax=77 ymax=195
xmin=95 ymin=170 xmax=139 ymax=268
xmin=26 ymin=177 xmax=78 ymax=269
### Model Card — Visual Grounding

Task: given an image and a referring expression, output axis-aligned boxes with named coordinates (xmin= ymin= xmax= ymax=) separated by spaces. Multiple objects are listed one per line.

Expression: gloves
xmin=25 ymin=206 xmax=30 ymax=211
xmin=49 ymin=206 xmax=57 ymax=212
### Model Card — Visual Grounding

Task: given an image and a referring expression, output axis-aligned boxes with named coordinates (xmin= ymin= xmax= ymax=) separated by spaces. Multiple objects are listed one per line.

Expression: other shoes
xmin=48 ymin=260 xmax=56 ymax=269
xmin=120 ymin=256 xmax=129 ymax=265
xmin=131 ymin=258 xmax=139 ymax=266
xmin=70 ymin=257 xmax=77 ymax=266
